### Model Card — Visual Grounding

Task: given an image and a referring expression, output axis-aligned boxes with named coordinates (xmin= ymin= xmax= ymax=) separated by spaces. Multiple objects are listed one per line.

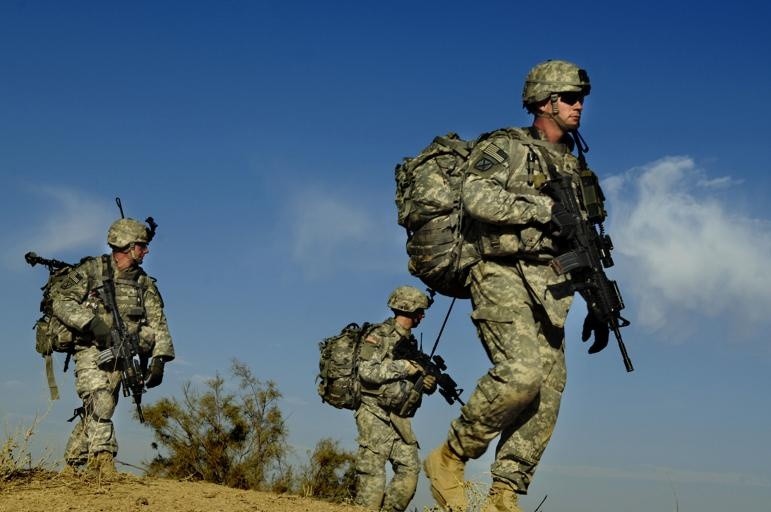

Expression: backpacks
xmin=317 ymin=321 xmax=369 ymax=410
xmin=37 ymin=268 xmax=85 ymax=357
xmin=395 ymin=131 xmax=477 ymax=298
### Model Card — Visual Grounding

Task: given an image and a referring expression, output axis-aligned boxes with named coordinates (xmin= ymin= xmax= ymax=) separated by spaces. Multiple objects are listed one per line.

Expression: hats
xmin=386 ymin=284 xmax=434 ymax=313
xmin=107 ymin=219 xmax=152 ymax=249
xmin=522 ymin=60 xmax=591 ymax=108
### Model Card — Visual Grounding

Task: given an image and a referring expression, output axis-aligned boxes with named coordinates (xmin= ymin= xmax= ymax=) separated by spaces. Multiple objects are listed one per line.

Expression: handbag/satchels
xmin=378 ymin=380 xmax=422 ymax=416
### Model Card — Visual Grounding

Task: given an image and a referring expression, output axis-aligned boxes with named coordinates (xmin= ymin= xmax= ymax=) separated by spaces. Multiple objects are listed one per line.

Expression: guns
xmin=536 ymin=174 xmax=636 ymax=372
xmin=391 ymin=334 xmax=466 ymax=410
xmin=88 ymin=281 xmax=152 ymax=425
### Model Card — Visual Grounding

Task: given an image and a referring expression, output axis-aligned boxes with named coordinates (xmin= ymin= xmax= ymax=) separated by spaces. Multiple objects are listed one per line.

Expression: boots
xmin=64 ymin=459 xmax=86 ymax=478
xmin=422 ymin=440 xmax=471 ymax=511
xmin=484 ymin=481 xmax=524 ymax=512
xmin=93 ymin=456 xmax=122 ymax=476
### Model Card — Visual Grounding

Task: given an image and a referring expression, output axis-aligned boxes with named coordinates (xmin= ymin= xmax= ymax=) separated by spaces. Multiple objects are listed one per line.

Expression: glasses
xmin=559 ymin=94 xmax=586 ymax=106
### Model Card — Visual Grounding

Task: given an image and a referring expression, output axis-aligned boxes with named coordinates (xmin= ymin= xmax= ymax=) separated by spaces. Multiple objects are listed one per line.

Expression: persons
xmin=354 ymin=287 xmax=438 ymax=511
xmin=423 ymin=59 xmax=610 ymax=511
xmin=48 ymin=217 xmax=176 ymax=474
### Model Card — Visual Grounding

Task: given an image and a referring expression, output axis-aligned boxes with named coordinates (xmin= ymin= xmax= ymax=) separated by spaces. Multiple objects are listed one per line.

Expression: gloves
xmin=581 ymin=311 xmax=609 ymax=354
xmin=406 ymin=359 xmax=426 ymax=377
xmin=423 ymin=374 xmax=438 ymax=395
xmin=145 ymin=355 xmax=166 ymax=390
xmin=89 ymin=316 xmax=115 ymax=347
xmin=549 ymin=203 xmax=578 ymax=248
